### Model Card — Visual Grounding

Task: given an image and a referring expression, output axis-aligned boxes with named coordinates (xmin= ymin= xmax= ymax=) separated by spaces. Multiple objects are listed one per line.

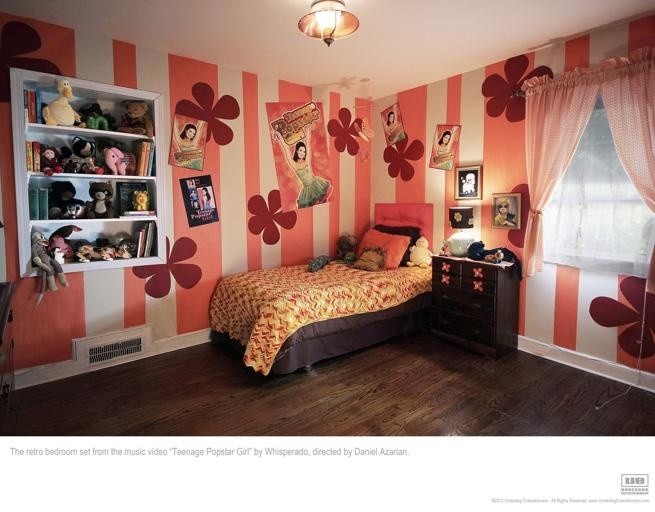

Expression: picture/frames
xmin=490 ymin=193 xmax=522 ymax=230
xmin=454 ymin=165 xmax=483 ymax=201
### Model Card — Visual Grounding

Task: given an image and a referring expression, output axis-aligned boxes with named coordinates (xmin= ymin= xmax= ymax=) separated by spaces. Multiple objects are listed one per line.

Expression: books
xmin=26 ymin=141 xmax=34 ymax=171
xmin=29 ymin=188 xmax=39 ymax=219
xmin=35 ymin=90 xmax=43 ymax=124
xmin=37 ymin=189 xmax=48 ymax=219
xmin=32 ymin=142 xmax=40 ymax=172
xmin=24 ymin=89 xmax=29 ymax=124
xmin=27 ymin=91 xmax=36 ymax=124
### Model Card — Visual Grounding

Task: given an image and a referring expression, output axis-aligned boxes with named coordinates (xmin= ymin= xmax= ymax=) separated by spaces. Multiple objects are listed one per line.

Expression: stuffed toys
xmin=305 ymin=254 xmax=331 ymax=272
xmin=31 ymin=231 xmax=68 ymax=306
xmin=407 ymin=237 xmax=433 ymax=269
xmin=335 ymin=233 xmax=359 ymax=260
xmin=43 ymin=78 xmax=155 ymax=263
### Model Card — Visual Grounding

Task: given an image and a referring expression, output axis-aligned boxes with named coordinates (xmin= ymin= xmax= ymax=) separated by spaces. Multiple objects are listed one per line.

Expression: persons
xmin=172 ymin=116 xmax=206 ymax=171
xmin=494 ymin=197 xmax=516 ymax=227
xmin=186 ymin=179 xmax=214 ymax=213
xmin=272 ymin=126 xmax=332 ymax=210
xmin=434 ymin=126 xmax=459 ymax=170
xmin=381 ymin=105 xmax=405 ymax=145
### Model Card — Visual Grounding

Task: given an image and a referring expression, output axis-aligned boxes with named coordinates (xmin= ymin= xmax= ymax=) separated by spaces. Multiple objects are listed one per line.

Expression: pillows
xmin=355 ymin=228 xmax=412 ymax=271
xmin=372 ymin=223 xmax=421 ymax=267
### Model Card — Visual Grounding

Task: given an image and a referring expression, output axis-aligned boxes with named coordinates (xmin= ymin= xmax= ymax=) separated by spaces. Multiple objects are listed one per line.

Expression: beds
xmin=220 ymin=202 xmax=436 ymax=377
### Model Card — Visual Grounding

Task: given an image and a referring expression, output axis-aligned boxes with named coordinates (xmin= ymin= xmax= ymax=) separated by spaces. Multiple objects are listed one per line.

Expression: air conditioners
xmin=71 ymin=323 xmax=154 ymax=371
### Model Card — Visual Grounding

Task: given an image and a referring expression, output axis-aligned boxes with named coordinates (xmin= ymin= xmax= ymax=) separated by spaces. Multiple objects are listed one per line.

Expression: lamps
xmin=297 ymin=0 xmax=362 ymax=48
xmin=447 ymin=206 xmax=474 ymax=256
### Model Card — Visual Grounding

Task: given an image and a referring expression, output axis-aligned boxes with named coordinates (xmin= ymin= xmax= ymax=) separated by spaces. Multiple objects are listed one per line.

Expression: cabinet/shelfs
xmin=429 ymin=253 xmax=520 ymax=361
xmin=0 ymin=280 xmax=16 ymax=436
xmin=8 ymin=64 xmax=168 ymax=280
